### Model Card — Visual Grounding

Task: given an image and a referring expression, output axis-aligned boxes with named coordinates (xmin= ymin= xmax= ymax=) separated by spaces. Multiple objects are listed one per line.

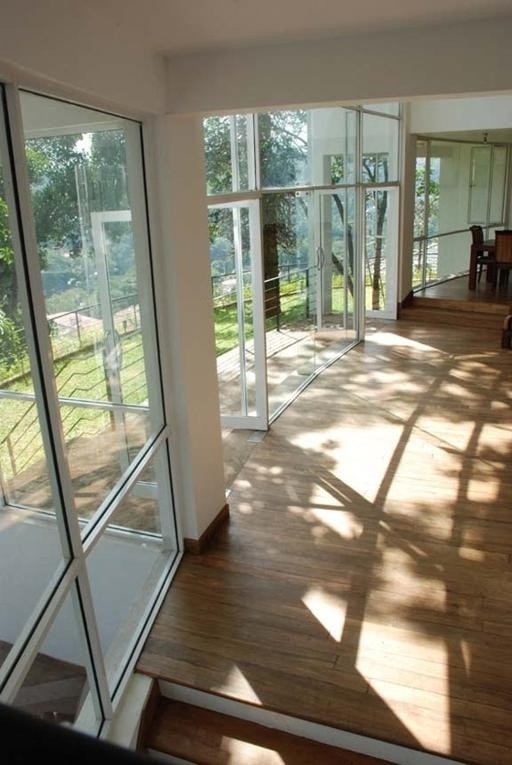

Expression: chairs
xmin=469 ymin=225 xmax=497 ymax=288
xmin=494 ymin=230 xmax=512 ymax=293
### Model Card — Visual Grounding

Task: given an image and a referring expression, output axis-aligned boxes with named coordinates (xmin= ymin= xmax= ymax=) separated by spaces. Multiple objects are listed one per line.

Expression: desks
xmin=476 ymin=239 xmax=495 ymax=281
